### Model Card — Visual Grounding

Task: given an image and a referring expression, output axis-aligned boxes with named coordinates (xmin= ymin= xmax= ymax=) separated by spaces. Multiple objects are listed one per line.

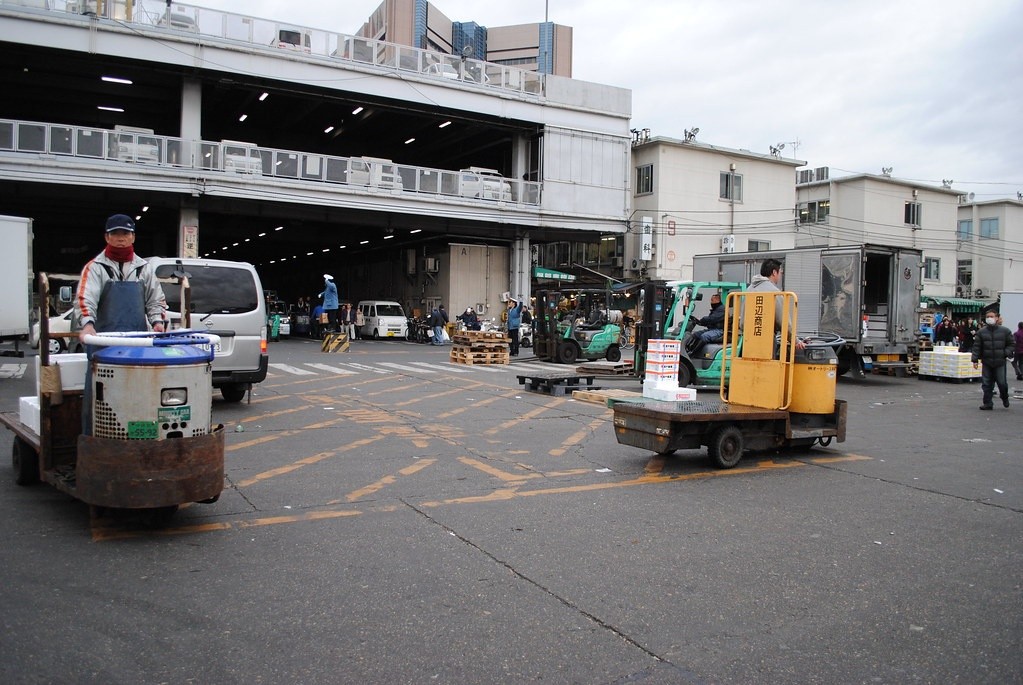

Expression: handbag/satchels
xmin=442 ymin=329 xmax=450 ymax=340
xmin=427 ymin=330 xmax=435 ymax=337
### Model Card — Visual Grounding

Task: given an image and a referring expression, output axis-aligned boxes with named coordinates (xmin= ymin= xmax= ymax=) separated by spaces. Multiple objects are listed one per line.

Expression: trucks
xmin=661 ymin=242 xmax=924 ymax=376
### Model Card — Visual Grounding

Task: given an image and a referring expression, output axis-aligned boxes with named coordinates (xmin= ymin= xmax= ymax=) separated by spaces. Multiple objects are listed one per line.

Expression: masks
xmin=986 ymin=317 xmax=996 ymax=325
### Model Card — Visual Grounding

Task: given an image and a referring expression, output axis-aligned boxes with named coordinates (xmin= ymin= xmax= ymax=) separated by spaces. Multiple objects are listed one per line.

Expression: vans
xmin=140 ymin=257 xmax=269 ymax=404
xmin=357 ymin=300 xmax=408 ymax=340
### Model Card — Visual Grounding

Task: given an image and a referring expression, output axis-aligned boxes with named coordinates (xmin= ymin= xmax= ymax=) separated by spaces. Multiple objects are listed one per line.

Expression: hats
xmin=467 ymin=308 xmax=471 ymax=312
xmin=510 ymin=298 xmax=517 ymax=304
xmin=439 ymin=304 xmax=444 ymax=308
xmin=324 ymin=274 xmax=334 ymax=281
xmin=106 ymin=214 xmax=135 ymax=232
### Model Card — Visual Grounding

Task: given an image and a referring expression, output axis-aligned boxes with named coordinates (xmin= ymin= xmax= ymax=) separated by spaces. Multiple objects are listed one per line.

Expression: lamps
xmin=943 ymin=179 xmax=956 ymax=191
xmin=770 ymin=143 xmax=785 ymax=157
xmin=684 ymin=127 xmax=699 ymax=142
xmin=460 ymin=45 xmax=473 ymax=62
xmin=882 ymin=166 xmax=893 ymax=177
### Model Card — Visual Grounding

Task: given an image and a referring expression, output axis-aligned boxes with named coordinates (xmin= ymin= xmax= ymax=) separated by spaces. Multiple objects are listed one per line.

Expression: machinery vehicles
xmin=509 ymin=288 xmax=623 ymax=364
xmin=594 ymin=281 xmax=751 ymax=388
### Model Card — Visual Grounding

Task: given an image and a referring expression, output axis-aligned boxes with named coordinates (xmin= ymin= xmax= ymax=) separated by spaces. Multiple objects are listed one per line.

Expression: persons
xmin=685 ymin=293 xmax=731 ymax=356
xmin=521 ymin=305 xmax=533 ymax=324
xmin=508 ymin=294 xmax=523 ymax=356
xmin=740 ymin=258 xmax=806 ymax=360
xmin=1011 ymin=322 xmax=1023 ymax=380
xmin=971 ymin=309 xmax=1016 ymax=410
xmin=318 ymin=274 xmax=339 ymax=333
xmin=296 ymin=294 xmax=325 ymax=338
xmin=272 ymin=305 xmax=281 ymax=312
xmin=933 ymin=315 xmax=987 ymax=352
xmin=337 ymin=302 xmax=365 ymax=341
xmin=74 ymin=215 xmax=167 ymax=437
xmin=459 ymin=307 xmax=481 ymax=330
xmin=428 ymin=304 xmax=450 ymax=346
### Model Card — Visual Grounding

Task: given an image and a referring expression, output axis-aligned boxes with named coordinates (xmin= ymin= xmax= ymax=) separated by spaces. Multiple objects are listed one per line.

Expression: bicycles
xmin=407 ymin=315 xmax=434 ymax=344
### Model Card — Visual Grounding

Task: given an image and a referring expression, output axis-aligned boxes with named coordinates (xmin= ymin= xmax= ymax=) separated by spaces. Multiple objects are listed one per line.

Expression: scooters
xmin=454 ymin=314 xmax=537 ymax=348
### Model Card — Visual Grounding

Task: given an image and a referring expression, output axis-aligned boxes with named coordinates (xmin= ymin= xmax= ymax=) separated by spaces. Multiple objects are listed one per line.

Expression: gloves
xmin=700 ymin=318 xmax=705 ymax=323
xmin=318 ymin=294 xmax=321 ymax=298
xmin=325 ymin=278 xmax=328 ymax=281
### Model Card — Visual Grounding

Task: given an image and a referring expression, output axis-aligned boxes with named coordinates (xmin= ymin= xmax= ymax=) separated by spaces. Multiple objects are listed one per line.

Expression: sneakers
xmin=1003 ymin=398 xmax=1010 ymax=407
xmin=980 ymin=404 xmax=993 ymax=410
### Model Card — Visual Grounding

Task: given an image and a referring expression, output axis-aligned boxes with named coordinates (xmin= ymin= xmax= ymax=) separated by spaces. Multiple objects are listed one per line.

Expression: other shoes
xmin=1017 ymin=375 xmax=1023 ymax=380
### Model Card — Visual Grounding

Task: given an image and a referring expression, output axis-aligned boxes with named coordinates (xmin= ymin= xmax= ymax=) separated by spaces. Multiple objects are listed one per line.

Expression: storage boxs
xmin=643 ymin=338 xmax=697 ymax=402
xmin=918 ymin=346 xmax=983 ymax=378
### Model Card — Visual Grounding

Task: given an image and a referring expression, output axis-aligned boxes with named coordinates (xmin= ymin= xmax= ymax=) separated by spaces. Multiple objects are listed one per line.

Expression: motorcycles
xmin=606 ymin=291 xmax=848 ymax=470
xmin=263 ymin=290 xmax=354 ymax=344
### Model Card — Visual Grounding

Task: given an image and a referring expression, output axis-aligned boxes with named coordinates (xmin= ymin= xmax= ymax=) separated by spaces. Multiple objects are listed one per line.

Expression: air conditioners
xmin=956 ymin=284 xmax=967 ymax=293
xmin=976 ymin=286 xmax=993 ymax=297
xmin=611 ymin=257 xmax=623 ymax=267
xmin=630 ymin=257 xmax=644 ymax=270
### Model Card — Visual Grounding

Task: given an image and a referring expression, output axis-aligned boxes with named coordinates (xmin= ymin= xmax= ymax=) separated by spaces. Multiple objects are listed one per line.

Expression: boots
xmin=688 ymin=340 xmax=706 ymax=355
xmin=688 ymin=335 xmax=700 ymax=351
